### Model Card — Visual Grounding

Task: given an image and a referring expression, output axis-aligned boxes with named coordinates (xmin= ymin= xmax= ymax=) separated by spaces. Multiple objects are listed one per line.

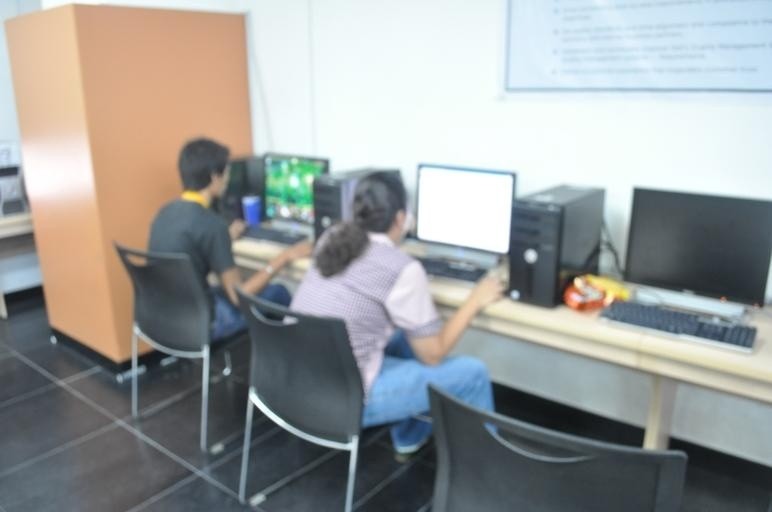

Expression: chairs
xmin=427 ymin=384 xmax=687 ymax=511
xmin=234 ymin=285 xmax=433 ymax=510
xmin=111 ymin=239 xmax=275 ymax=455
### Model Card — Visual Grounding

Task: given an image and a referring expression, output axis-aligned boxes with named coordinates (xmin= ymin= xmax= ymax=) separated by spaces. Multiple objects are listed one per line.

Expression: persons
xmin=281 ymin=170 xmax=506 ymax=454
xmin=147 ymin=137 xmax=312 ymax=342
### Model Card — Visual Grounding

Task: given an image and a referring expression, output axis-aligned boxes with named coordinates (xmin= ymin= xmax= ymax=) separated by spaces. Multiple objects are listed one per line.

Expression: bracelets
xmin=264 ymin=265 xmax=277 ymax=277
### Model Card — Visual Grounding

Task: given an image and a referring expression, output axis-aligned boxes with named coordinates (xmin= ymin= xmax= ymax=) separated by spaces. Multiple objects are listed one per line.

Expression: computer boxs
xmin=507 ymin=183 xmax=605 ymax=309
xmin=312 ymin=166 xmax=401 ymax=243
xmin=213 ymin=154 xmax=272 ymax=226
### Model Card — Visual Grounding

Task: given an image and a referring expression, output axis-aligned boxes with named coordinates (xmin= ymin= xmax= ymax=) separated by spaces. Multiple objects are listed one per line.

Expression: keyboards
xmin=241 ymin=226 xmax=308 ymax=247
xmin=597 ymin=297 xmax=759 ymax=354
xmin=412 ymin=255 xmax=487 ymax=282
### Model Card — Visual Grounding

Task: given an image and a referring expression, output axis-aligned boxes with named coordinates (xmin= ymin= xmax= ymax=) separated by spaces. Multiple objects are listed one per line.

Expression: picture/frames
xmin=505 ymin=1 xmax=772 ymax=92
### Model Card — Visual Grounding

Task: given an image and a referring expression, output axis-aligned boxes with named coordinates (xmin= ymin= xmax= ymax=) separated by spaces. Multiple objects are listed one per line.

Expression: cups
xmin=241 ymin=197 xmax=262 ymax=229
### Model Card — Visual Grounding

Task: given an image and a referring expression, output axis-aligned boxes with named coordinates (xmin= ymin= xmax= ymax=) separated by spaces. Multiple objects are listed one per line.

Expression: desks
xmin=230 ymin=219 xmax=772 ymax=449
xmin=0 ymin=209 xmax=44 ymax=321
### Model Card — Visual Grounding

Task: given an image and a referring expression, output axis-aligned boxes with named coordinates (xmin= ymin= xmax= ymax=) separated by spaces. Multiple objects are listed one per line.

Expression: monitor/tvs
xmin=622 ymin=186 xmax=772 ymax=320
xmin=414 ymin=163 xmax=517 ymax=266
xmin=261 ymin=152 xmax=331 ymax=235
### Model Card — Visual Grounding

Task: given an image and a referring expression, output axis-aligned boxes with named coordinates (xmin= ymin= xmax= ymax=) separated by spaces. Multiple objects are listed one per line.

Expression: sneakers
xmin=390 ymin=424 xmax=431 ymax=453
